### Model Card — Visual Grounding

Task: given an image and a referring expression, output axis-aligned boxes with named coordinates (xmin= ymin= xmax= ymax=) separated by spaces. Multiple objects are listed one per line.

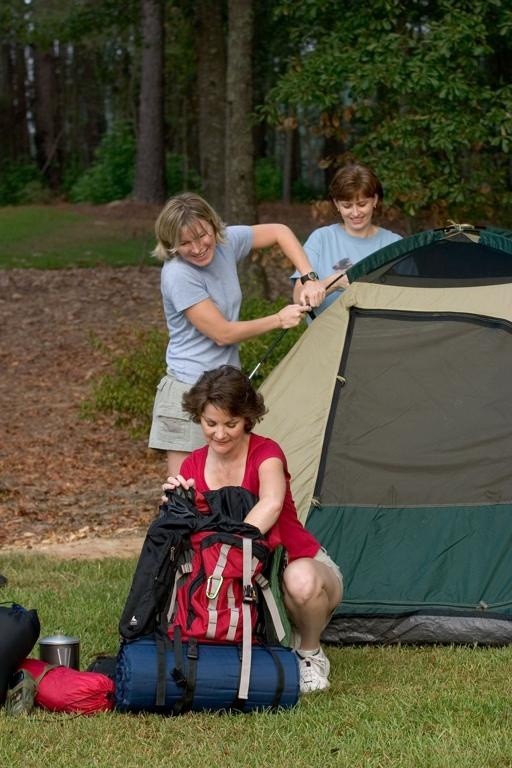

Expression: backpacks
xmin=119 ymin=485 xmax=291 ymax=649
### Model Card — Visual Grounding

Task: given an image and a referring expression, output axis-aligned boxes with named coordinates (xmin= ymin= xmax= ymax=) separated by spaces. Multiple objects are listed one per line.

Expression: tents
xmin=246 ymin=223 xmax=511 ymax=647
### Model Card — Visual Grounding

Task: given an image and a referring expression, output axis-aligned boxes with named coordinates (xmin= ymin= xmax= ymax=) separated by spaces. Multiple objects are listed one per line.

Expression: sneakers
xmin=295 ymin=647 xmax=330 ymax=694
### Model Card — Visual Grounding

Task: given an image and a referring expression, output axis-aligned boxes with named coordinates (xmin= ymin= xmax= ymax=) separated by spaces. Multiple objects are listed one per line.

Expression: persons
xmin=149 ymin=193 xmax=325 ymax=476
xmin=289 ymin=164 xmax=403 ymax=326
xmin=158 ymin=365 xmax=343 ymax=694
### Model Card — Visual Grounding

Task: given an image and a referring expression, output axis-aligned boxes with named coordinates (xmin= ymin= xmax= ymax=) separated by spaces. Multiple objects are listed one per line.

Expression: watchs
xmin=301 ymin=272 xmax=319 ymax=285
